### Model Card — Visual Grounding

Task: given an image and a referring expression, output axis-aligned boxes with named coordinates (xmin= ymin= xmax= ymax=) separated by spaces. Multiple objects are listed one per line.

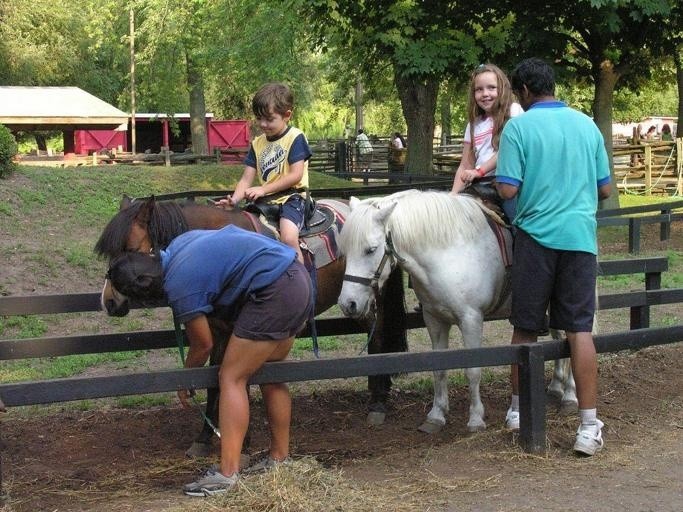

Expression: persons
xmin=495 ymin=58 xmax=611 ymax=457
xmin=647 ymin=126 xmax=656 ymax=140
xmin=408 ymin=63 xmax=525 ymax=314
xmin=354 ymin=129 xmax=374 ymax=173
xmin=662 ymin=124 xmax=672 ymax=141
xmin=216 ymin=83 xmax=312 ymax=266
xmin=392 ymin=132 xmax=406 ymax=148
xmin=112 ymin=223 xmax=315 ymax=496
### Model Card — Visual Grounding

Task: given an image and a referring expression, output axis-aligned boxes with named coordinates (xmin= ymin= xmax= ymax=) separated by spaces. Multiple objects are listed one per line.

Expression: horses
xmin=94 ymin=194 xmax=407 ymax=467
xmin=337 ymin=188 xmax=599 ymax=434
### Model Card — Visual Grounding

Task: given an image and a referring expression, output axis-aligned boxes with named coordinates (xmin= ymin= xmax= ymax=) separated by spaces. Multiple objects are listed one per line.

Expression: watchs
xmin=476 ymin=165 xmax=484 ymax=176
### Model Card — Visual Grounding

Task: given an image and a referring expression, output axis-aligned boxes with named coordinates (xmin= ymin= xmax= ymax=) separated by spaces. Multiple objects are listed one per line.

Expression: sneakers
xmin=502 ymin=408 xmax=520 ymax=430
xmin=243 ymin=455 xmax=293 ymax=477
xmin=574 ymin=419 xmax=604 ymax=455
xmin=183 ymin=465 xmax=240 ymax=495
